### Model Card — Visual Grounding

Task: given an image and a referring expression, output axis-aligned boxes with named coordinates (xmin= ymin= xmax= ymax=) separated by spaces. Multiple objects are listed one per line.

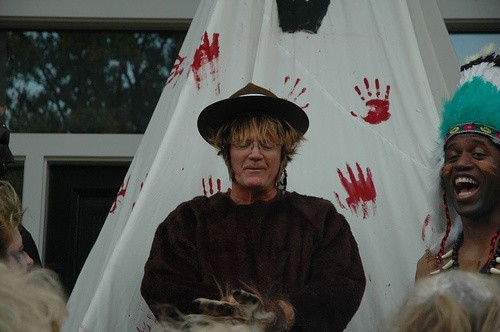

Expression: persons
xmin=0 ymin=122 xmax=70 ymax=332
xmin=391 ymin=269 xmax=500 ymax=332
xmin=140 ymin=88 xmax=366 ymax=332
xmin=413 ymin=112 xmax=500 ymax=285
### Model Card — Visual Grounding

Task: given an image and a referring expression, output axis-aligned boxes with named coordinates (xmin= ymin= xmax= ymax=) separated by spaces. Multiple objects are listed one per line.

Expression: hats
xmin=196 ymin=83 xmax=309 ymax=146
xmin=440 ymin=49 xmax=500 ymax=144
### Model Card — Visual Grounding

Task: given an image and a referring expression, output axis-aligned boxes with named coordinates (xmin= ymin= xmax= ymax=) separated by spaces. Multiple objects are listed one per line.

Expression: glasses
xmin=230 ymin=140 xmax=282 ymax=151
